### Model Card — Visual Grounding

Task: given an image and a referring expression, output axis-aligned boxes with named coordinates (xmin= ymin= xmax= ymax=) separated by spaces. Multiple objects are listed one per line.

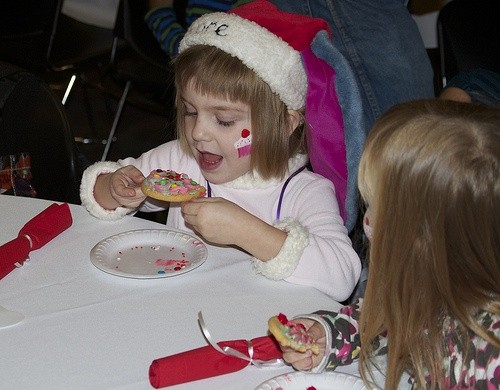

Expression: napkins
xmin=0 ymin=203 xmax=73 ymax=279
xmin=148 ymin=335 xmax=284 ymax=389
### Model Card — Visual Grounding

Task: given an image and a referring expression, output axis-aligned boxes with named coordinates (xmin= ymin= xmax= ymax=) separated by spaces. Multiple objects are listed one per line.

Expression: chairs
xmin=0 ymin=73 xmax=81 ymax=205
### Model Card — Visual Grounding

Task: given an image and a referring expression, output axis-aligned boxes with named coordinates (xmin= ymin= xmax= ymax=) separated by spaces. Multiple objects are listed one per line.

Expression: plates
xmin=89 ymin=228 xmax=209 ymax=279
xmin=254 ymin=370 xmax=383 ymax=390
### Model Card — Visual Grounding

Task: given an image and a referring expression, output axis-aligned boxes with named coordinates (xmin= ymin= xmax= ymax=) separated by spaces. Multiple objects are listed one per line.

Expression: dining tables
xmin=0 ymin=193 xmax=416 ymax=390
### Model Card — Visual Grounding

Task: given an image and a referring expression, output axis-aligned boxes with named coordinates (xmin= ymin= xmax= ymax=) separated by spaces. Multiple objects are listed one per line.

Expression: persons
xmin=79 ymin=0 xmax=362 ymax=303
xmin=143 ymin=0 xmax=500 ymax=199
xmin=276 ymin=99 xmax=500 ymax=390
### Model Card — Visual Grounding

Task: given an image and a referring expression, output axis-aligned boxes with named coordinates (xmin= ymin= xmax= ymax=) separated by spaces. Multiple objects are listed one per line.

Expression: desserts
xmin=141 ymin=168 xmax=206 ymax=202
xmin=267 ymin=313 xmax=320 ymax=354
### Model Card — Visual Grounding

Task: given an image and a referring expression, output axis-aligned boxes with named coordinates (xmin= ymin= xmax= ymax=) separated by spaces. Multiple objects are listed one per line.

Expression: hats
xmin=177 ymin=0 xmax=365 ymax=234
